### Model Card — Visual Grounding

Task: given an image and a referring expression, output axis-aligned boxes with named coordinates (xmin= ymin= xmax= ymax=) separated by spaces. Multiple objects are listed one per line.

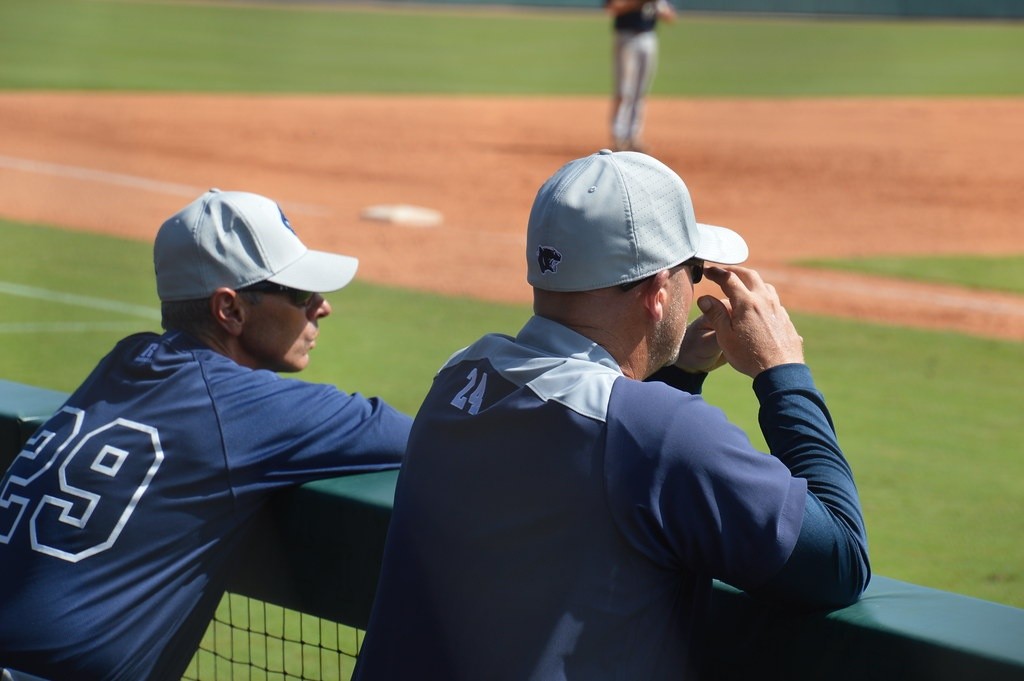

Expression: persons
xmin=350 ymin=148 xmax=872 ymax=681
xmin=0 ymin=188 xmax=415 ymax=681
xmin=604 ymin=0 xmax=674 ymax=156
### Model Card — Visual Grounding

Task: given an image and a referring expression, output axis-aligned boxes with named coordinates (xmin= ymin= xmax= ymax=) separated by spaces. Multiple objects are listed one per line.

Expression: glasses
xmin=622 ymin=258 xmax=704 ymax=292
xmin=250 ymin=282 xmax=313 ymax=309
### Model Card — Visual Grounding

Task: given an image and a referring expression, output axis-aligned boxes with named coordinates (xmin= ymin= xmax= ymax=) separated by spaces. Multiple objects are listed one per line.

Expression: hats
xmin=527 ymin=149 xmax=748 ymax=292
xmin=153 ymin=189 xmax=361 ymax=301
